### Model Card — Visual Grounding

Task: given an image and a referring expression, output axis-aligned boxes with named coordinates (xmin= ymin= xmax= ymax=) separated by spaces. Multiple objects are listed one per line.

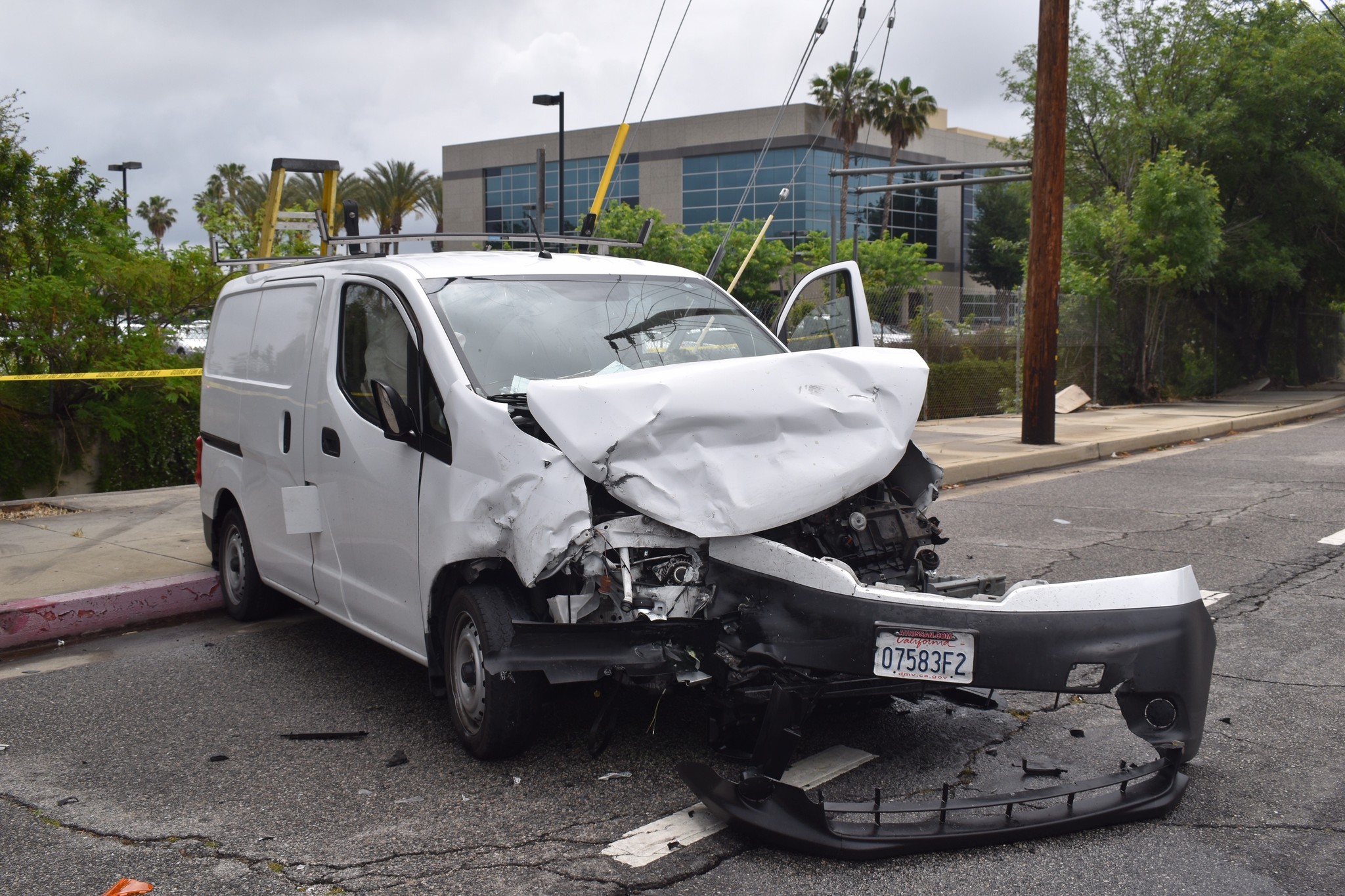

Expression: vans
xmin=195 ymin=232 xmax=1219 ymax=860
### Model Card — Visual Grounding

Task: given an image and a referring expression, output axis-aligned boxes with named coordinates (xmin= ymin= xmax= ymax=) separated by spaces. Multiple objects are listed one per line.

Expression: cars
xmin=56 ymin=314 xmax=210 ymax=360
xmin=638 ymin=311 xmax=1019 ymax=355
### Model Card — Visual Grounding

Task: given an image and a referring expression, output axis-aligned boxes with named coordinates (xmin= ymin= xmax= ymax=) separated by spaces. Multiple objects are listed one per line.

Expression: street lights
xmin=533 ymin=91 xmax=564 ymax=253
xmin=108 ymin=161 xmax=142 ymax=336
